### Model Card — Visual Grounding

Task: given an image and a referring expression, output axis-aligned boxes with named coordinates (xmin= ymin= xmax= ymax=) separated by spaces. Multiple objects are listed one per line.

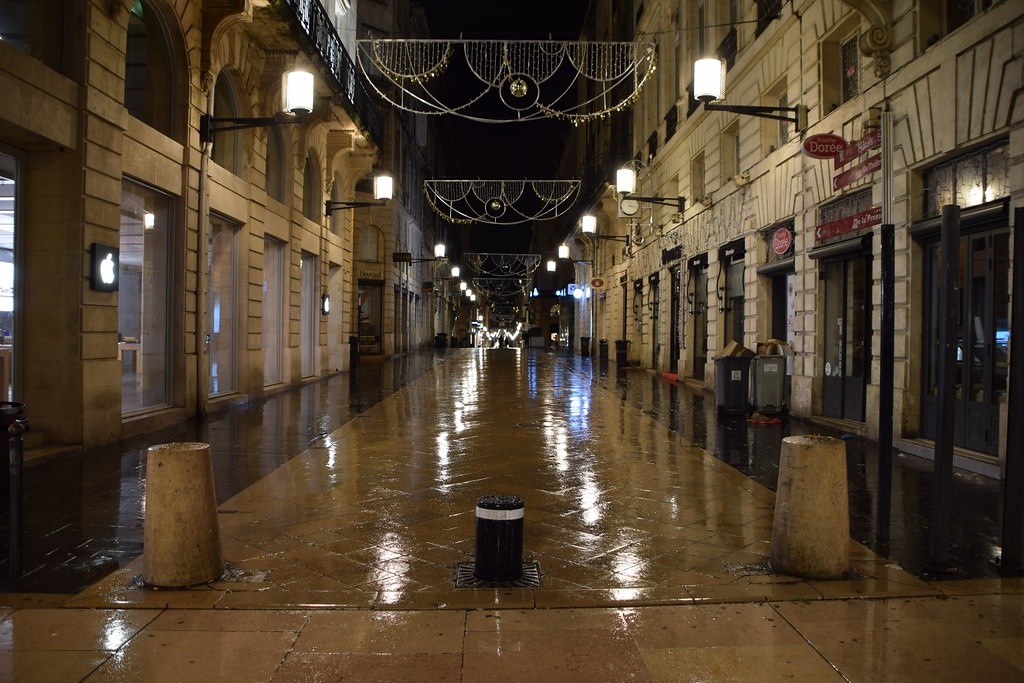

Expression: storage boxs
xmin=720 ymin=339 xmax=747 ymax=357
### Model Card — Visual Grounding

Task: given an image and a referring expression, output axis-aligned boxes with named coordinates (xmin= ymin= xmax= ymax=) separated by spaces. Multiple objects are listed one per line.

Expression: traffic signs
xmin=832 ymin=151 xmax=881 ymax=191
xmin=815 ymin=206 xmax=883 ymax=240
xmin=833 ymin=127 xmax=881 ymax=170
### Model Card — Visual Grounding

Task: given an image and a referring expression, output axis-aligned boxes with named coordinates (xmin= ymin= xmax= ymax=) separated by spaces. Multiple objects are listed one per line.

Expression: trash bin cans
xmin=580 ymin=337 xmax=590 ymax=356
xmin=0 ymin=401 xmax=32 ymax=504
xmin=754 ymin=354 xmax=789 ymax=416
xmin=711 ymin=356 xmax=753 ymax=417
xmin=599 ymin=339 xmax=608 ymax=360
xmin=614 ymin=340 xmax=630 ymax=367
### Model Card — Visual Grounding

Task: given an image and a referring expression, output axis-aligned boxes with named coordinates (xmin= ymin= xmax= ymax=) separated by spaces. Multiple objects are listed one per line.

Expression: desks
xmin=117 ymin=342 xmax=142 ymax=375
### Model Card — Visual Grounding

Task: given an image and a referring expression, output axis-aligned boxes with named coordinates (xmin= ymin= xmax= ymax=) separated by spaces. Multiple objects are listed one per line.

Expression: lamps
xmin=324 ymin=176 xmax=393 ymax=217
xmin=199 ymin=72 xmax=316 ymax=141
xmin=547 ymin=260 xmax=576 ymax=272
xmin=409 ymin=244 xmax=447 ymax=266
xmin=617 ymin=169 xmax=686 ymax=212
xmin=583 ymin=215 xmax=630 ymax=245
xmin=435 ymin=267 xmax=460 ymax=282
xmin=451 ymin=283 xmax=476 ymax=302
xmin=694 ymin=57 xmax=808 ymax=131
xmin=559 ymin=245 xmax=594 ymax=266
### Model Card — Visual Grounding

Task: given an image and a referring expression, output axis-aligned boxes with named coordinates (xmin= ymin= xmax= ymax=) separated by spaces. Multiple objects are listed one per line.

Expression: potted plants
xmin=529 ymin=327 xmax=546 ymax=347
